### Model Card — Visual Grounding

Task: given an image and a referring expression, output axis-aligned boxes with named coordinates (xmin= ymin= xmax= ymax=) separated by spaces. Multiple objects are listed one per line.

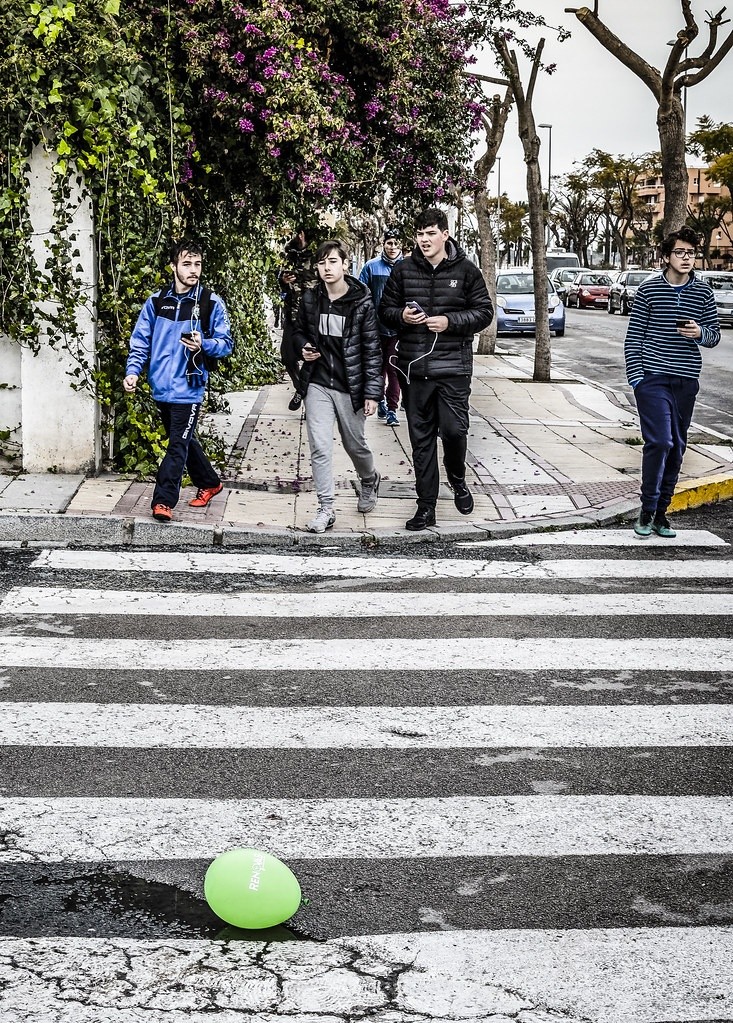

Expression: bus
xmin=546 ymin=247 xmax=580 ymax=279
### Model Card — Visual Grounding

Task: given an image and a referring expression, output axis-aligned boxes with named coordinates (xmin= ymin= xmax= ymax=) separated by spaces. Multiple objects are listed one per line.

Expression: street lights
xmin=572 ymin=160 xmax=590 ymax=230
xmin=538 ymin=123 xmax=552 ymax=248
xmin=667 ymin=40 xmax=690 ymax=135
xmin=496 ymin=157 xmax=501 ymax=269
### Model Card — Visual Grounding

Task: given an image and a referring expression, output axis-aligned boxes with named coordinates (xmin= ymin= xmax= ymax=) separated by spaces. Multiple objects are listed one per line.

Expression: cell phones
xmin=677 ymin=320 xmax=691 ymax=332
xmin=406 ymin=302 xmax=429 ymax=318
xmin=180 ymin=332 xmax=192 ymax=344
xmin=304 ymin=347 xmax=319 ymax=353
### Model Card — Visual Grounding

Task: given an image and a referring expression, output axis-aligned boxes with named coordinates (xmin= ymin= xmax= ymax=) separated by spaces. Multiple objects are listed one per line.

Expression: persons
xmin=267 ymin=248 xmax=321 ymax=420
xmin=624 ymin=226 xmax=721 ymax=538
xmin=123 ymin=242 xmax=234 ymax=522
xmin=376 ymin=208 xmax=494 ymax=531
xmin=292 ymin=240 xmax=384 ymax=534
xmin=358 ymin=230 xmax=405 ymax=426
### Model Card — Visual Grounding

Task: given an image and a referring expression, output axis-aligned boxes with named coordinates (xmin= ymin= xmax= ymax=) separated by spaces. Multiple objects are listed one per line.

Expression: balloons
xmin=205 ymin=849 xmax=310 ymax=928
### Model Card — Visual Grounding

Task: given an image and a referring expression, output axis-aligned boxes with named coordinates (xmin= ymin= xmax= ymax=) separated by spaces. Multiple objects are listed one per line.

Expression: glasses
xmin=670 ymin=250 xmax=695 ymax=258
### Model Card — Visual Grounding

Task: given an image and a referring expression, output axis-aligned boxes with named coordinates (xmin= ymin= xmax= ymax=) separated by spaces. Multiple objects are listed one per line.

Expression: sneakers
xmin=357 ymin=471 xmax=381 ymax=513
xmin=190 ymin=482 xmax=221 ymax=508
xmin=377 ymin=401 xmax=387 ymax=418
xmin=151 ymin=502 xmax=173 ymax=522
xmin=650 ymin=521 xmax=677 ymax=538
xmin=288 ymin=392 xmax=302 ymax=411
xmin=309 ymin=507 xmax=335 ymax=534
xmin=455 ymin=481 xmax=473 ymax=514
xmin=404 ymin=507 xmax=437 ymax=531
xmin=385 ymin=411 xmax=400 ymax=426
xmin=632 ymin=520 xmax=651 ymax=535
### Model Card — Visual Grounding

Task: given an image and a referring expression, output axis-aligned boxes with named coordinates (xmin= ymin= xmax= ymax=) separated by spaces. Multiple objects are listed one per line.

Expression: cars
xmin=608 ymin=270 xmax=657 ymax=316
xmin=550 ymin=267 xmax=592 ymax=307
xmin=694 ymin=270 xmax=733 ymax=327
xmin=495 ymin=269 xmax=566 ymax=337
xmin=566 ymin=272 xmax=613 ymax=310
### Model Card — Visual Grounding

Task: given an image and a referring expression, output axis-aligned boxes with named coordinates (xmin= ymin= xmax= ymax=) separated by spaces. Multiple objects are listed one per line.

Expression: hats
xmin=384 ymin=228 xmax=404 ymax=240
